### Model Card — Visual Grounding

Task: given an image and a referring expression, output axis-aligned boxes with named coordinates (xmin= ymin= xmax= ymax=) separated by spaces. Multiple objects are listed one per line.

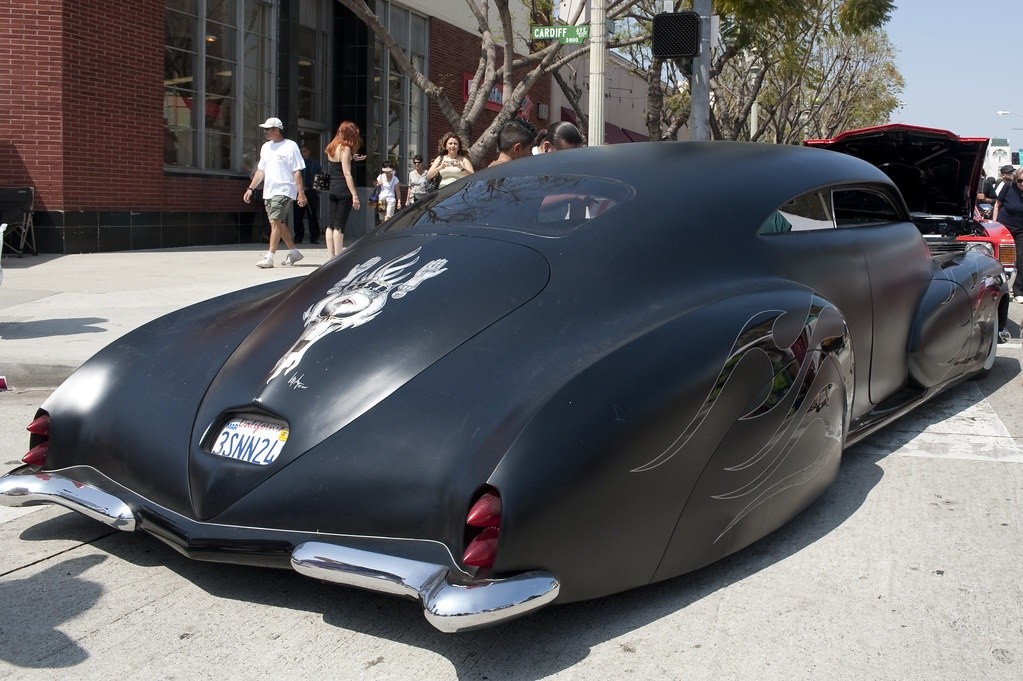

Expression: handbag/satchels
xmin=377 ymin=201 xmax=386 ymax=211
xmin=427 ymin=154 xmax=443 ymax=192
xmin=369 ymin=175 xmax=382 ymax=203
xmin=313 ymin=158 xmax=330 ymax=191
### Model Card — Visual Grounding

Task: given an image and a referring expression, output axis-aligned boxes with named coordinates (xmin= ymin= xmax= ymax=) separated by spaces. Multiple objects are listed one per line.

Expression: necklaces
xmin=446 ymin=154 xmax=458 ymax=159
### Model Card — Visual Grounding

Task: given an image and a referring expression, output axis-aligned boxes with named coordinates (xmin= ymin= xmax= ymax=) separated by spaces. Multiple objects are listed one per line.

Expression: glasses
xmin=413 ymin=162 xmax=420 ymax=164
xmin=1016 ymin=179 xmax=1023 ymax=183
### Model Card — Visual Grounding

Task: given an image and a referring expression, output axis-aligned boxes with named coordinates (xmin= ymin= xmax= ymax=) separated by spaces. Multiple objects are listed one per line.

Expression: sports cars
xmin=1 ymin=140 xmax=1009 ymax=634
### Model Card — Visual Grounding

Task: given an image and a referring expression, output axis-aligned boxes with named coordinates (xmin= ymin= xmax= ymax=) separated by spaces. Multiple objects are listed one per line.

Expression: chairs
xmin=0 ymin=187 xmax=39 ymax=259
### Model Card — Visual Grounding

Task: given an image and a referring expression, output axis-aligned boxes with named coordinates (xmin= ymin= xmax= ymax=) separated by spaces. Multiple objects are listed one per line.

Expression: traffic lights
xmin=652 ymin=12 xmax=701 ymax=56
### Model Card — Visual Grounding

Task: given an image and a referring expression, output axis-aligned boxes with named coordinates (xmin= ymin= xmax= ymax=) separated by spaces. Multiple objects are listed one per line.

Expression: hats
xmin=382 ymin=167 xmax=393 ymax=172
xmin=1001 ymin=165 xmax=1016 ymax=174
xmin=259 ymin=117 xmax=283 ymax=130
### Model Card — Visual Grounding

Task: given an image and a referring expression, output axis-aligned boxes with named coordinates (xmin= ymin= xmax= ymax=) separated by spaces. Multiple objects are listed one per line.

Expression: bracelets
xmin=461 ymin=168 xmax=465 ymax=171
xmin=247 ymin=187 xmax=253 ymax=191
xmin=353 ymin=193 xmax=359 ymax=198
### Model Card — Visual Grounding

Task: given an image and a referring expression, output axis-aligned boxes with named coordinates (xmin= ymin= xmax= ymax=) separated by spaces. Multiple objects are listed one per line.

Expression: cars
xmin=803 ymin=124 xmax=1018 ymax=305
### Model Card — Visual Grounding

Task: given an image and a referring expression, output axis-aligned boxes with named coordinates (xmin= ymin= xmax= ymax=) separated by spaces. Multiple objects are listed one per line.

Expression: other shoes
xmin=1013 ymin=295 xmax=1023 ymax=304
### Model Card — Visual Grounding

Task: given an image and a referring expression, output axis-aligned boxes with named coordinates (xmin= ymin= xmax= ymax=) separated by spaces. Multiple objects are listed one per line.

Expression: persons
xmin=323 ymin=121 xmax=367 ymax=259
xmin=977 ymin=165 xmax=1023 ymax=304
xmin=405 ymin=154 xmax=430 ymax=207
xmin=426 ymin=132 xmax=475 ymax=190
xmin=300 ymin=144 xmax=322 ymax=245
xmin=375 ymin=160 xmax=402 ymax=224
xmin=484 ymin=116 xmax=617 ymax=223
xmin=243 ymin=117 xmax=308 ymax=268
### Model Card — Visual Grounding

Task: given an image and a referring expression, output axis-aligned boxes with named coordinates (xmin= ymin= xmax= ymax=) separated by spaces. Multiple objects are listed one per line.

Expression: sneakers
xmin=256 ymin=257 xmax=274 ymax=268
xmin=281 ymin=251 xmax=303 ymax=265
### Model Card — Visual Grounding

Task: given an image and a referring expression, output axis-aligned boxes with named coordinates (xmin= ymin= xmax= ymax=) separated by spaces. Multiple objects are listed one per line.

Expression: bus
xmin=982 ymin=137 xmax=1023 ymax=197
